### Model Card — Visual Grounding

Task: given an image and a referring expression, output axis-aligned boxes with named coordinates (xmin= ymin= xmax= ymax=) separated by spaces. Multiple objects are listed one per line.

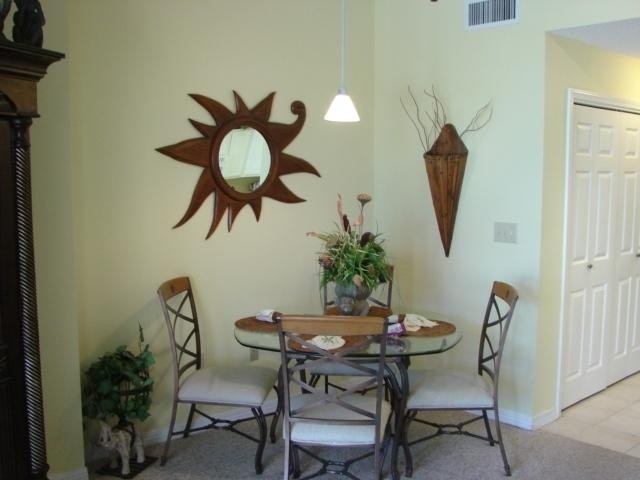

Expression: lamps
xmin=324 ymin=0 xmax=360 ymax=122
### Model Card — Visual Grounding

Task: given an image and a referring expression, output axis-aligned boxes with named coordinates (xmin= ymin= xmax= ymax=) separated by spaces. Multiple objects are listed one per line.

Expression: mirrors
xmin=155 ymin=89 xmax=321 ymax=240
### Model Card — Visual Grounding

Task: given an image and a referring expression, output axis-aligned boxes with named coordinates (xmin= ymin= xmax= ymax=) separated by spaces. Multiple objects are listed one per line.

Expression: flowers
xmin=305 ymin=192 xmax=394 ymax=296
